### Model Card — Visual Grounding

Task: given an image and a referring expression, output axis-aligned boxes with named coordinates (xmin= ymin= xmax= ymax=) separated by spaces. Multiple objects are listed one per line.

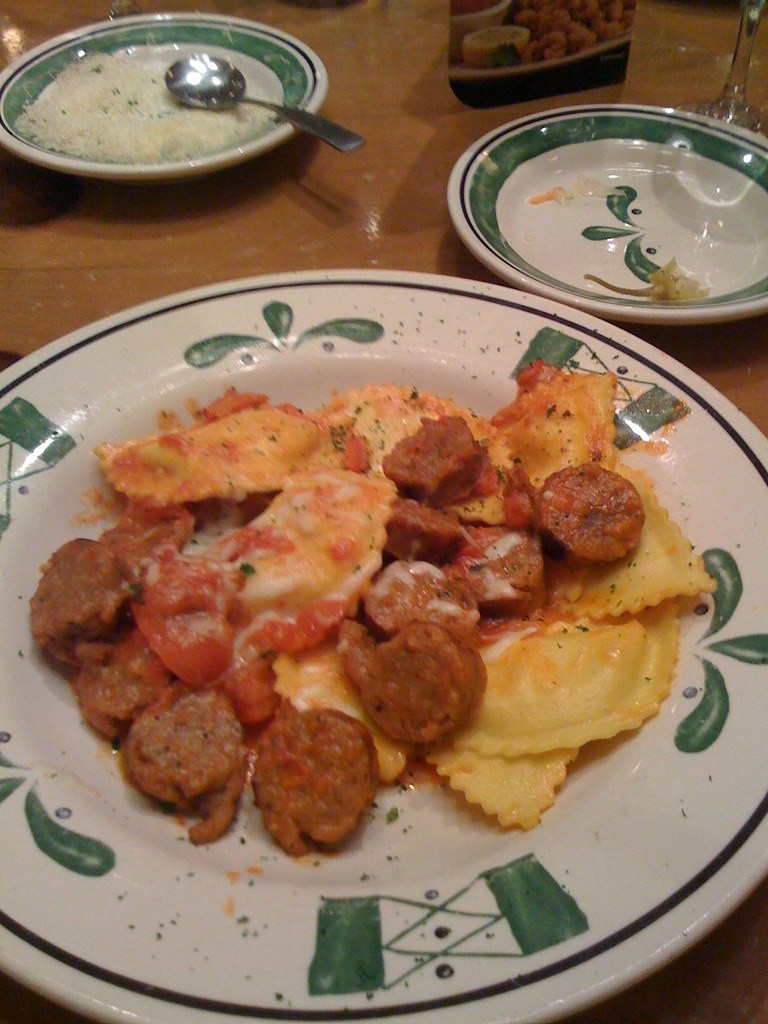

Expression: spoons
xmin=165 ymin=53 xmax=365 ymax=155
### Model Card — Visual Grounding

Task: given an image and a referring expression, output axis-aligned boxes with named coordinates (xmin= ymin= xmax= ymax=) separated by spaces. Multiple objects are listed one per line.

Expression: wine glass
xmin=674 ymin=0 xmax=768 ymax=134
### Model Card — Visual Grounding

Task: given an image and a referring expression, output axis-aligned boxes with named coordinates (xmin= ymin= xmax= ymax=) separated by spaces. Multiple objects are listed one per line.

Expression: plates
xmin=0 ymin=13 xmax=328 ymax=186
xmin=448 ymin=104 xmax=768 ymax=326
xmin=0 ymin=268 xmax=768 ymax=1023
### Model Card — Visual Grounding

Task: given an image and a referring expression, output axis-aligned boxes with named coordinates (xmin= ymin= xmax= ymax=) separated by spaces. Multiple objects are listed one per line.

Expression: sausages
xmin=28 ymin=410 xmax=644 ymax=856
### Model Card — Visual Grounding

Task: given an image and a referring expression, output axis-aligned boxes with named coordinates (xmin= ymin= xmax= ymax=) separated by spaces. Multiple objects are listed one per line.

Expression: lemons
xmin=463 ymin=24 xmax=531 ymax=72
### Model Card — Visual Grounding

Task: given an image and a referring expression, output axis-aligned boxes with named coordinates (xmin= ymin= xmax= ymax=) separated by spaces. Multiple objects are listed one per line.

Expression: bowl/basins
xmin=448 ymin=0 xmax=631 ymax=109
